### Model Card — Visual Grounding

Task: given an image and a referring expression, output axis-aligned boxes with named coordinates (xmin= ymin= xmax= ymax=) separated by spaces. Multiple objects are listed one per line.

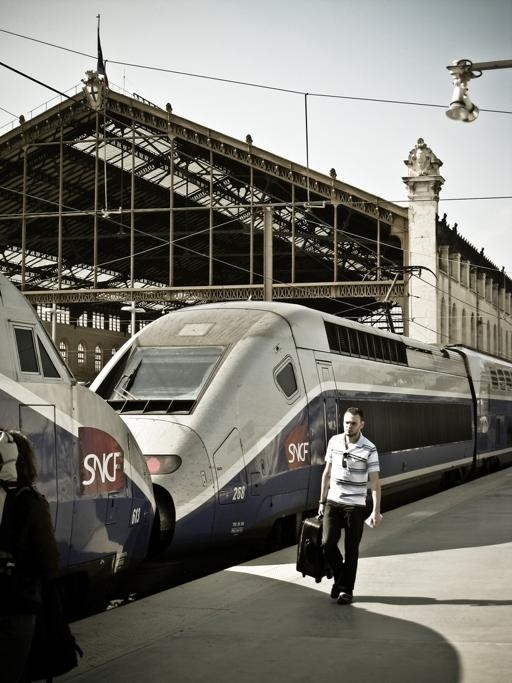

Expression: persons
xmin=317 ymin=407 xmax=380 ymax=604
xmin=1 ymin=428 xmax=84 ymax=680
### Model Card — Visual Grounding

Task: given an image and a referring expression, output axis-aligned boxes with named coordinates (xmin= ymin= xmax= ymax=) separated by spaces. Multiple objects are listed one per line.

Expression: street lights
xmin=445 ymin=59 xmax=512 ymax=122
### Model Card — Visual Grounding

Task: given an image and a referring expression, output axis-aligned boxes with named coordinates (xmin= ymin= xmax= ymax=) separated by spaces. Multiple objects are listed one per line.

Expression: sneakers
xmin=337 ymin=591 xmax=353 ymax=604
xmin=331 ymin=583 xmax=341 ymax=598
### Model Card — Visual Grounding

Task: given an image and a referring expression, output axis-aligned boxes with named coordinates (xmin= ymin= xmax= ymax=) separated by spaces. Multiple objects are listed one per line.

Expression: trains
xmin=0 ymin=271 xmax=512 ymax=590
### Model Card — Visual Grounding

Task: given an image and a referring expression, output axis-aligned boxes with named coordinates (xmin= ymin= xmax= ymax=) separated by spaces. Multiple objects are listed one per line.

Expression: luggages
xmin=296 ymin=513 xmax=334 ymax=583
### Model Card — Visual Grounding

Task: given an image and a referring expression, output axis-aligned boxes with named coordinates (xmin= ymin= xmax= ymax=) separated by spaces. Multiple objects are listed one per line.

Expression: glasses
xmin=342 ymin=452 xmax=348 ymax=468
